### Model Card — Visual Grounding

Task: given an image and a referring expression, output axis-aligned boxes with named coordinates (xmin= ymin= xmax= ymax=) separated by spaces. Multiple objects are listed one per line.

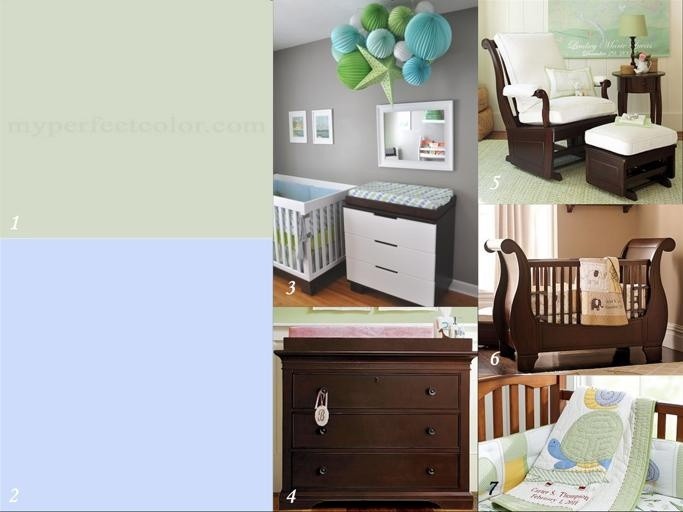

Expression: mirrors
xmin=375 ymin=100 xmax=456 ymax=171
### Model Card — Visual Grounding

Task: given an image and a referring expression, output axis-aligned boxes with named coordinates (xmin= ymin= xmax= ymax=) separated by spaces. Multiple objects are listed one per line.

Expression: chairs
xmin=481 ymin=28 xmax=617 ymax=182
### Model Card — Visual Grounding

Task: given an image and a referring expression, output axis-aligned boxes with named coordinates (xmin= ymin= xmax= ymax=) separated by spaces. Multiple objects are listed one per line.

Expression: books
xmin=615 ymin=112 xmax=650 ymax=128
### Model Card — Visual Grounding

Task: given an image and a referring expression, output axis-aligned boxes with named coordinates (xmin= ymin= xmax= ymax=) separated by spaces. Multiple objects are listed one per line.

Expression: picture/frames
xmin=288 ymin=110 xmax=308 ymax=144
xmin=311 ymin=109 xmax=334 ymax=145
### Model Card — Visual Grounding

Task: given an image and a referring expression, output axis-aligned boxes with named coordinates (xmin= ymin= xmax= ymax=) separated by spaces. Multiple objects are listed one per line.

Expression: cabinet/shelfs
xmin=273 ymin=337 xmax=479 ymax=510
xmin=417 ymin=135 xmax=445 ymax=161
xmin=341 ymin=195 xmax=457 ymax=307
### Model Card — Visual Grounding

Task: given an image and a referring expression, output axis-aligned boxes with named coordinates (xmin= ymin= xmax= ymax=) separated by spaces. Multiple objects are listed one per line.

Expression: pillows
xmin=544 ymin=65 xmax=597 ymax=100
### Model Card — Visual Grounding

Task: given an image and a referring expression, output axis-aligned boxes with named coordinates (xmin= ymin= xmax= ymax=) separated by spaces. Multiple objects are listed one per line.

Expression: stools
xmin=583 ymin=122 xmax=678 ymax=201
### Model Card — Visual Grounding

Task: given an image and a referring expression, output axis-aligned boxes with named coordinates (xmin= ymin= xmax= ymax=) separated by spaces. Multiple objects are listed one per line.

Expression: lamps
xmin=619 ymin=14 xmax=648 ymax=68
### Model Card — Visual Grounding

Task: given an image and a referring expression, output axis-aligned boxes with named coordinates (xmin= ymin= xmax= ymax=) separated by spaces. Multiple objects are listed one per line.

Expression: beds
xmin=478 ymin=374 xmax=683 ymax=512
xmin=273 ymin=173 xmax=359 ymax=295
xmin=612 ymin=71 xmax=666 ymax=126
xmin=484 ymin=237 xmax=676 ymax=374
